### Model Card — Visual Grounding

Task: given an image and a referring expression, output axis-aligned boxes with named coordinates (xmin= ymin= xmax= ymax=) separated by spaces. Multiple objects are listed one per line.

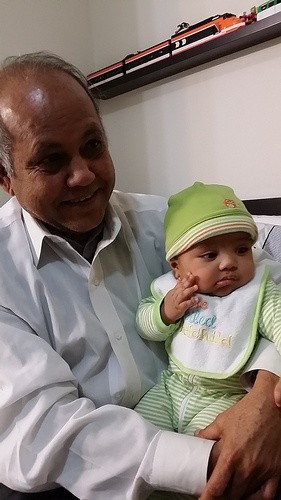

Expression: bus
xmin=255 ymin=0 xmax=280 ymax=22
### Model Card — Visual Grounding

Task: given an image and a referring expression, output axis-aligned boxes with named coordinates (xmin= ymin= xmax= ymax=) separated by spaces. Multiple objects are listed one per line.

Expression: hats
xmin=164 ymin=182 xmax=259 ymax=262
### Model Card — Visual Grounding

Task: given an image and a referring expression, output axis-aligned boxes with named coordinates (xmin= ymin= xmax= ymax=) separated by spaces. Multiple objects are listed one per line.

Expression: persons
xmin=0 ymin=49 xmax=281 ymax=500
xmin=130 ymin=183 xmax=281 ymax=440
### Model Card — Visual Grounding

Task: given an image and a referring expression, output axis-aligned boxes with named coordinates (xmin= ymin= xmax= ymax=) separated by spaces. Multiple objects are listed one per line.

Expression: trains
xmin=85 ymin=13 xmax=247 ymax=91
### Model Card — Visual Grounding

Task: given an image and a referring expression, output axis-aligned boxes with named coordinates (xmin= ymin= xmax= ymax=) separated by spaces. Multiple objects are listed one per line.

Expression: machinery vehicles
xmin=239 ymin=7 xmax=257 ymax=25
